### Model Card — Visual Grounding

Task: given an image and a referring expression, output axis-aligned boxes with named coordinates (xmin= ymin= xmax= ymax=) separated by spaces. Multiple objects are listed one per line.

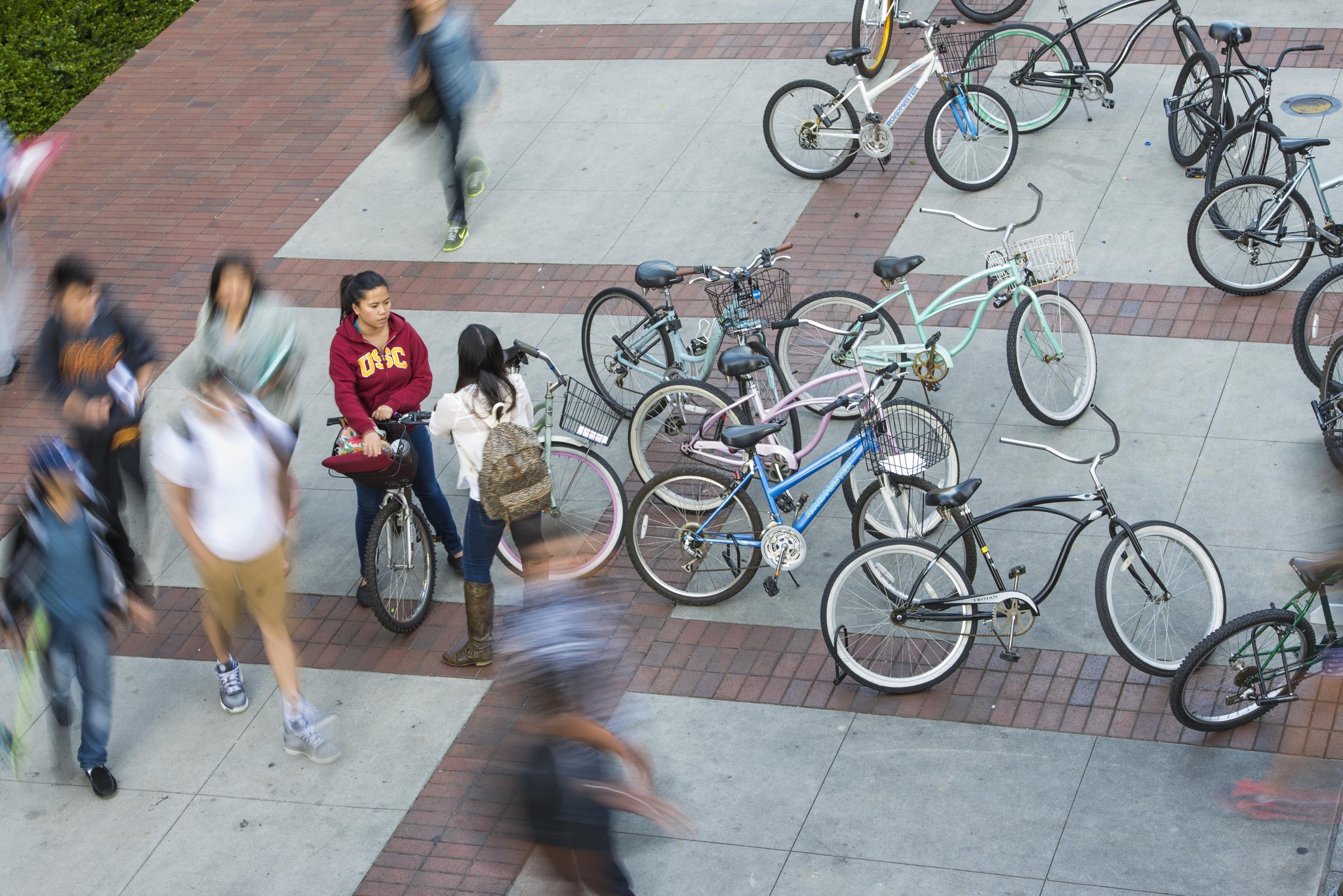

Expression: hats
xmin=35 ymin=444 xmax=82 ymax=472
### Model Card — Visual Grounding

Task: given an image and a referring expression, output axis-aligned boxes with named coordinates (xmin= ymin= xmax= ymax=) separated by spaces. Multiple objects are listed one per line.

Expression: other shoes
xmin=51 ymin=692 xmax=75 ymax=728
xmin=84 ymin=764 xmax=117 ymax=800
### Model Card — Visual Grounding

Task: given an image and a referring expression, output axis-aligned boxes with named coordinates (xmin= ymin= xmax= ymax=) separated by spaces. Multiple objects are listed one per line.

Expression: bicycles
xmin=1169 ymin=550 xmax=1343 ymax=734
xmin=1186 ymin=136 xmax=1343 ymax=296
xmin=851 ymin=0 xmax=1029 ymax=79
xmin=326 ymin=409 xmax=442 ymax=634
xmin=961 ymin=1 xmax=1236 ymax=136
xmin=1292 ymin=262 xmax=1343 ymax=472
xmin=1162 ymin=20 xmax=1326 ymax=244
xmin=625 ymin=361 xmax=978 ymax=607
xmin=626 ymin=310 xmax=962 ymax=540
xmin=494 ymin=337 xmax=630 ymax=586
xmin=762 ymin=17 xmax=1019 ymax=192
xmin=817 ymin=401 xmax=1229 ymax=697
xmin=581 ymin=240 xmax=802 ymax=481
xmin=774 ymin=181 xmax=1097 ymax=426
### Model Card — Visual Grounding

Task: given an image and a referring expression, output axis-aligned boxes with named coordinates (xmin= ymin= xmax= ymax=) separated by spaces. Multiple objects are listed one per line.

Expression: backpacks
xmin=456 ymin=393 xmax=553 ymax=522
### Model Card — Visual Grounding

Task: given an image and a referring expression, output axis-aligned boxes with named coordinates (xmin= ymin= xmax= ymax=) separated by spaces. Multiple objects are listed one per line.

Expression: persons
xmin=329 ymin=270 xmax=465 ymax=608
xmin=430 ymin=323 xmax=550 ymax=666
xmin=0 ymin=435 xmax=159 ymax=800
xmin=149 ymin=249 xmax=343 ymax=764
xmin=34 ymin=256 xmax=161 ymax=606
xmin=0 ymin=118 xmax=28 ymax=386
xmin=390 ymin=0 xmax=498 ymax=251
xmin=489 ymin=531 xmax=685 ymax=896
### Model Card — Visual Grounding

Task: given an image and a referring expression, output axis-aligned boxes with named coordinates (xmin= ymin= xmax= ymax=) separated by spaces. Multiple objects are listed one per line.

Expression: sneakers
xmin=443 ymin=225 xmax=468 ymax=252
xmin=466 ymin=168 xmax=484 ymax=197
xmin=215 ymin=657 xmax=248 ymax=714
xmin=283 ymin=719 xmax=342 ymax=765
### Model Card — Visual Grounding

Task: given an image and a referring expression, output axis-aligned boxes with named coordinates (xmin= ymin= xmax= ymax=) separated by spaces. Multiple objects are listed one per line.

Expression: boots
xmin=521 ymin=560 xmax=550 ymax=614
xmin=443 ymin=581 xmax=495 ymax=667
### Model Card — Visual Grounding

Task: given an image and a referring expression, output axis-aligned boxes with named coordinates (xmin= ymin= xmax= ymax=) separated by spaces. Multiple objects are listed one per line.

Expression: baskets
xmin=703 ymin=268 xmax=793 ymax=337
xmin=559 ymin=377 xmax=626 ymax=446
xmin=983 ymin=230 xmax=1079 ymax=295
xmin=931 ymin=30 xmax=998 ymax=76
xmin=855 ymin=397 xmax=954 ymax=476
